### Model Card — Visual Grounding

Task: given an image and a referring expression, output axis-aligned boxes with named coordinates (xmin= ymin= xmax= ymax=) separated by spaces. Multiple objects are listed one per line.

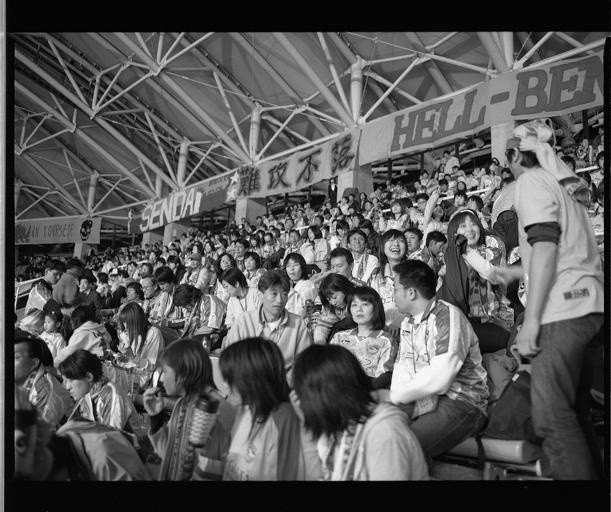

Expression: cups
xmin=184 ymin=393 xmax=222 ymax=448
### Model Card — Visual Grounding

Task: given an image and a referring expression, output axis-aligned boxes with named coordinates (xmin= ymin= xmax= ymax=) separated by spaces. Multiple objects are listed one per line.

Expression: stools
xmin=446 ymin=435 xmax=543 ymax=480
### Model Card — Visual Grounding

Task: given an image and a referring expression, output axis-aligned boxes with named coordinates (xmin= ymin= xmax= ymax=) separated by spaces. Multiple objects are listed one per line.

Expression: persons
xmin=15 ymin=264 xmax=238 ymax=481
xmin=220 ymin=258 xmax=489 ymax=481
xmin=486 ymin=118 xmax=604 ymax=482
xmin=21 ymin=145 xmax=515 ymax=356
